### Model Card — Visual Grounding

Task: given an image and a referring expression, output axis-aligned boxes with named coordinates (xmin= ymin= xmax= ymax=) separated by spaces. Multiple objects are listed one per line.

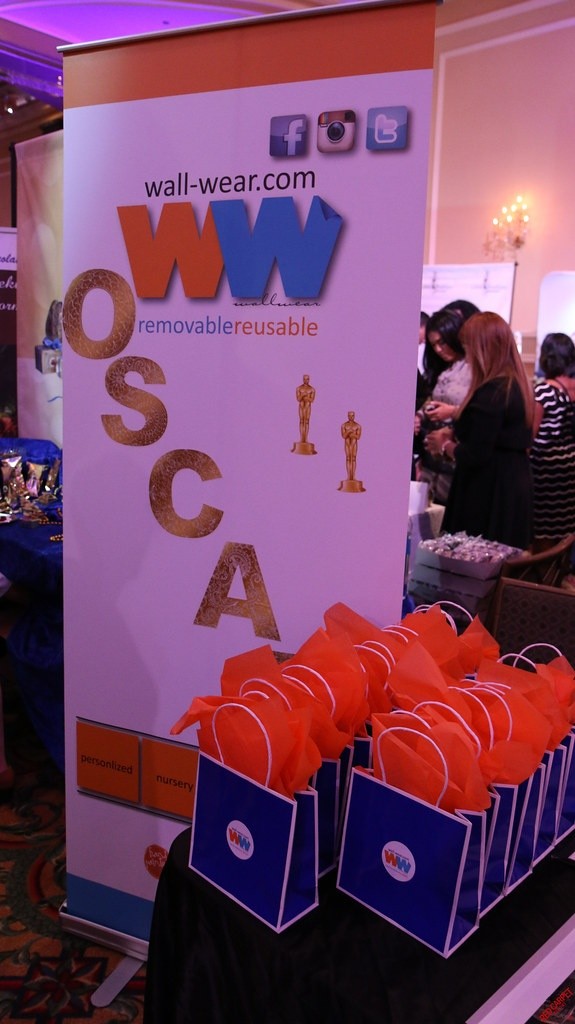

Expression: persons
xmin=411 ymin=300 xmax=575 ymax=552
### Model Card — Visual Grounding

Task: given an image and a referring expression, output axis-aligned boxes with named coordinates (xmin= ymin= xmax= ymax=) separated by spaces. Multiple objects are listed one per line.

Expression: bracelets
xmin=451 ymin=404 xmax=458 ymax=419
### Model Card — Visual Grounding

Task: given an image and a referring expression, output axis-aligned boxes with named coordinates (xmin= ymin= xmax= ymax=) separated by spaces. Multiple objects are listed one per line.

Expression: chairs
xmin=416 ymin=532 xmax=575 ymax=625
xmin=491 ymin=576 xmax=575 ymax=674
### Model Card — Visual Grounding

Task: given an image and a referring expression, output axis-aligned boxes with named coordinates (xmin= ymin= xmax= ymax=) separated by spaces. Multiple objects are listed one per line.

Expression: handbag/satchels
xmin=186 ymin=600 xmax=575 ymax=959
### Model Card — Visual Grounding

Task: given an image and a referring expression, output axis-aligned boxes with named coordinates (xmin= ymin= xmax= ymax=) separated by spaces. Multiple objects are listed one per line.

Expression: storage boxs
xmin=410 ymin=548 xmax=521 ymax=614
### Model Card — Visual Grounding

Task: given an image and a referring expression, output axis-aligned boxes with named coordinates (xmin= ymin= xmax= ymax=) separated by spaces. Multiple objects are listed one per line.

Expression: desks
xmin=145 ymin=823 xmax=575 ymax=1024
xmin=0 ymin=520 xmax=63 ymax=772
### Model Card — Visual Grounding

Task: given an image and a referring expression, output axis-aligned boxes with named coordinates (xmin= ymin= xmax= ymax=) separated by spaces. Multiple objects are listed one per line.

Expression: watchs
xmin=438 ymin=440 xmax=451 ymax=457
